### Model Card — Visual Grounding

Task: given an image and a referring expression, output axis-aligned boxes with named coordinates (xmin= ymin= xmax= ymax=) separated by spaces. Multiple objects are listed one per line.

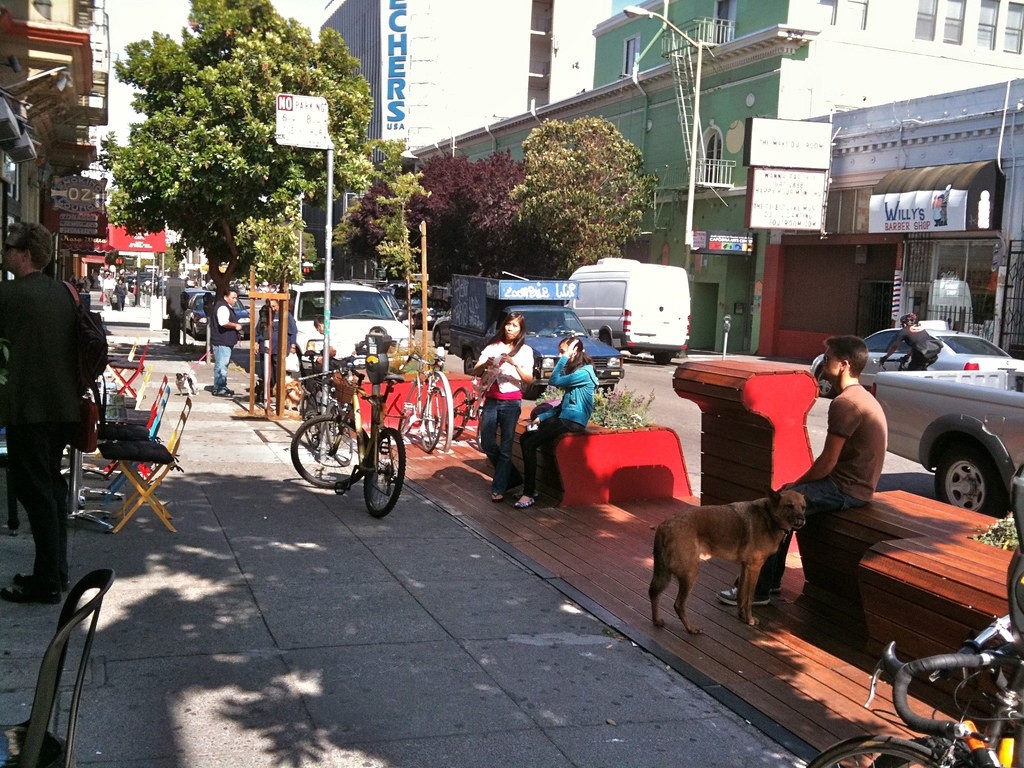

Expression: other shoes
xmin=514 ymin=490 xmax=539 ymax=500
xmin=514 ymin=497 xmax=536 ymax=508
xmin=211 ymin=390 xmax=234 ymax=397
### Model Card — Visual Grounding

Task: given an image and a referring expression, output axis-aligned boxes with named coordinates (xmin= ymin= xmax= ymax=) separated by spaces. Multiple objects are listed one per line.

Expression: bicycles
xmin=301 ymin=350 xmax=353 ymax=467
xmin=805 ymin=463 xmax=1024 ymax=768
xmin=292 ymin=355 xmax=406 ymax=518
xmin=398 ymin=351 xmax=484 ymax=456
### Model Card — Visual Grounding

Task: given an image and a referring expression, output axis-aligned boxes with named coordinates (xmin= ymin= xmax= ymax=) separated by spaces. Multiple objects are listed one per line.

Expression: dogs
xmin=276 ymin=375 xmax=304 ymax=411
xmin=175 ymin=372 xmax=199 ymax=397
xmin=648 ymin=486 xmax=807 ymax=636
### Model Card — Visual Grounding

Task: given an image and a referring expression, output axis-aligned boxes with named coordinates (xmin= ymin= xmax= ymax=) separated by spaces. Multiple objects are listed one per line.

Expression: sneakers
xmin=715 ymin=586 xmax=771 ymax=607
xmin=769 ymin=583 xmax=783 ymax=595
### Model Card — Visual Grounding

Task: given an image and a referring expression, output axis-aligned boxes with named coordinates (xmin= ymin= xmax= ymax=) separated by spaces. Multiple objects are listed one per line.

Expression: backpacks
xmin=62 ymin=280 xmax=109 ymax=432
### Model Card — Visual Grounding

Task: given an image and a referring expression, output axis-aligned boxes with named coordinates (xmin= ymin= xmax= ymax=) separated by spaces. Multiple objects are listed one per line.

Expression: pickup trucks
xmin=448 ymin=274 xmax=624 ymax=397
xmin=872 ymin=367 xmax=1024 ymax=519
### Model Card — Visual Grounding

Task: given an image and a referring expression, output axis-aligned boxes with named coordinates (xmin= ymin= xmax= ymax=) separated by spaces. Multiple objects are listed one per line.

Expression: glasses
xmin=3 ymin=243 xmax=25 ymax=253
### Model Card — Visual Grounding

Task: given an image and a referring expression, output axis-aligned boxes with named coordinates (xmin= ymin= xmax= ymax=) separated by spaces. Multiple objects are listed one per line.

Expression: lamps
xmin=0 ymin=54 xmax=23 ymax=74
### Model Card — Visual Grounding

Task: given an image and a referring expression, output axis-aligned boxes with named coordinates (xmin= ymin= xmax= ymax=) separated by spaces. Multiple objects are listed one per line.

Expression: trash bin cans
xmin=78 ymin=292 xmax=90 ymax=310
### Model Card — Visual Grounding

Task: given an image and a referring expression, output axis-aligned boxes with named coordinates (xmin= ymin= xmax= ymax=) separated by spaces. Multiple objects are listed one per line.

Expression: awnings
xmin=61 ymin=240 xmax=115 ymax=253
xmin=868 ymin=158 xmax=1005 ymax=232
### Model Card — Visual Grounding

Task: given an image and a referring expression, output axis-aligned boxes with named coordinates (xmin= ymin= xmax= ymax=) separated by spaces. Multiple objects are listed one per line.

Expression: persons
xmin=716 ymin=336 xmax=887 ymax=605
xmin=474 ymin=311 xmax=534 ymax=503
xmin=948 ymin=318 xmax=952 ymax=330
xmin=514 ymin=338 xmax=598 ymax=507
xmin=206 ymin=280 xmax=218 ymax=290
xmin=212 ymin=288 xmax=242 ymax=397
xmin=256 ymin=299 xmax=365 ymax=399
xmin=0 ymin=223 xmax=87 ymax=603
xmin=98 ymin=274 xmax=128 ymax=311
xmin=880 ymin=312 xmax=934 ymax=371
xmin=70 ymin=272 xmax=95 ymax=293
xmin=539 ymin=316 xmax=558 ymax=335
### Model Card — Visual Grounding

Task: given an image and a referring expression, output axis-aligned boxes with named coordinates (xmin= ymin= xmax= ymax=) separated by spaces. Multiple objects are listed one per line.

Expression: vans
xmin=562 ymin=258 xmax=691 ymax=365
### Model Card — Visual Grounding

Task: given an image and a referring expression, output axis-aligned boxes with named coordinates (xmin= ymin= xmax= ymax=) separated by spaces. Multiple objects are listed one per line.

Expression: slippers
xmin=491 ymin=492 xmax=503 ymax=502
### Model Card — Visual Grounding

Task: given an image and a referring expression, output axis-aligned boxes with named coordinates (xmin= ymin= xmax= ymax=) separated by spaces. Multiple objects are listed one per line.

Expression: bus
xmin=200 ymin=266 xmax=224 ymax=290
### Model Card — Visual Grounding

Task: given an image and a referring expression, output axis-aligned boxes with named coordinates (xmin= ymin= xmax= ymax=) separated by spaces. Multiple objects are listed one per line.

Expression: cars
xmin=234 ymin=279 xmax=258 ymax=298
xmin=277 ymin=281 xmax=415 ymax=373
xmin=383 ymin=282 xmax=451 ymax=347
xmin=128 ymin=272 xmax=159 ymax=293
xmin=810 ymin=329 xmax=1024 ymax=399
xmin=186 ymin=293 xmax=251 ymax=340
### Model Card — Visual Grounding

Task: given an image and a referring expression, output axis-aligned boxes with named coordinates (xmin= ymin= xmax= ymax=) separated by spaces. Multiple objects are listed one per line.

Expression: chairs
xmin=0 ymin=568 xmax=118 ymax=768
xmin=97 ymin=334 xmax=195 ymax=536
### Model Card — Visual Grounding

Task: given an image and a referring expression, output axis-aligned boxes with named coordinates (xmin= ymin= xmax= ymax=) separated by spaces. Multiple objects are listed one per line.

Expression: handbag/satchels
xmin=99 ymin=293 xmax=108 ymax=302
xmin=915 ymin=336 xmax=944 ymax=360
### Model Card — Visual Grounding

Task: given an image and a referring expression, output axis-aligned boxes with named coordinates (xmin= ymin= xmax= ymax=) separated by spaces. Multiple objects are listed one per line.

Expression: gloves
xmin=904 ymin=354 xmax=910 ymax=360
xmin=880 ymin=356 xmax=888 ymax=363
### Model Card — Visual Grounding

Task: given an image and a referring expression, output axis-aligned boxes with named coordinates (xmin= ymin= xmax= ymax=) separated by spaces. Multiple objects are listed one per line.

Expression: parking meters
xmin=180 ymin=292 xmax=189 ymax=345
xmin=365 ymin=326 xmax=392 ymax=439
xmin=203 ymin=292 xmax=214 ymax=363
xmin=722 ymin=314 xmax=732 ymax=359
xmin=259 ymin=305 xmax=275 ymax=411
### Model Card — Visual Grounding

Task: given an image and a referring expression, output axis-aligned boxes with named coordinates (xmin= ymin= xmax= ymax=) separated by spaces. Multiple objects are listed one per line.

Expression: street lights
xmin=623 ymin=7 xmax=703 ymax=244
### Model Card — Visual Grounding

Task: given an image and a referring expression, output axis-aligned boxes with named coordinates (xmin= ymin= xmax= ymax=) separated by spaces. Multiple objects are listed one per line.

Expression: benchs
xmin=331 ymin=370 xmax=479 ymax=431
xmin=495 ymin=405 xmax=694 ymax=507
xmin=810 ymin=490 xmax=1024 ymax=674
